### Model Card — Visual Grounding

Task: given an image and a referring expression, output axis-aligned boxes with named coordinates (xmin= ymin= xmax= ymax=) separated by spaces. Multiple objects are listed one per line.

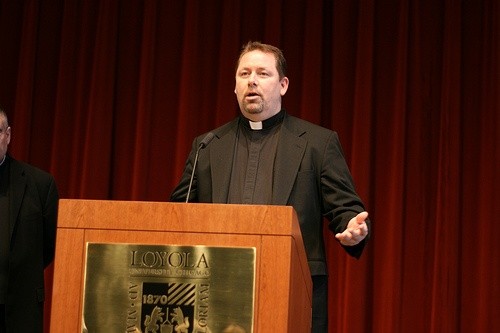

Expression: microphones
xmin=186 ymin=132 xmax=215 ymax=202
